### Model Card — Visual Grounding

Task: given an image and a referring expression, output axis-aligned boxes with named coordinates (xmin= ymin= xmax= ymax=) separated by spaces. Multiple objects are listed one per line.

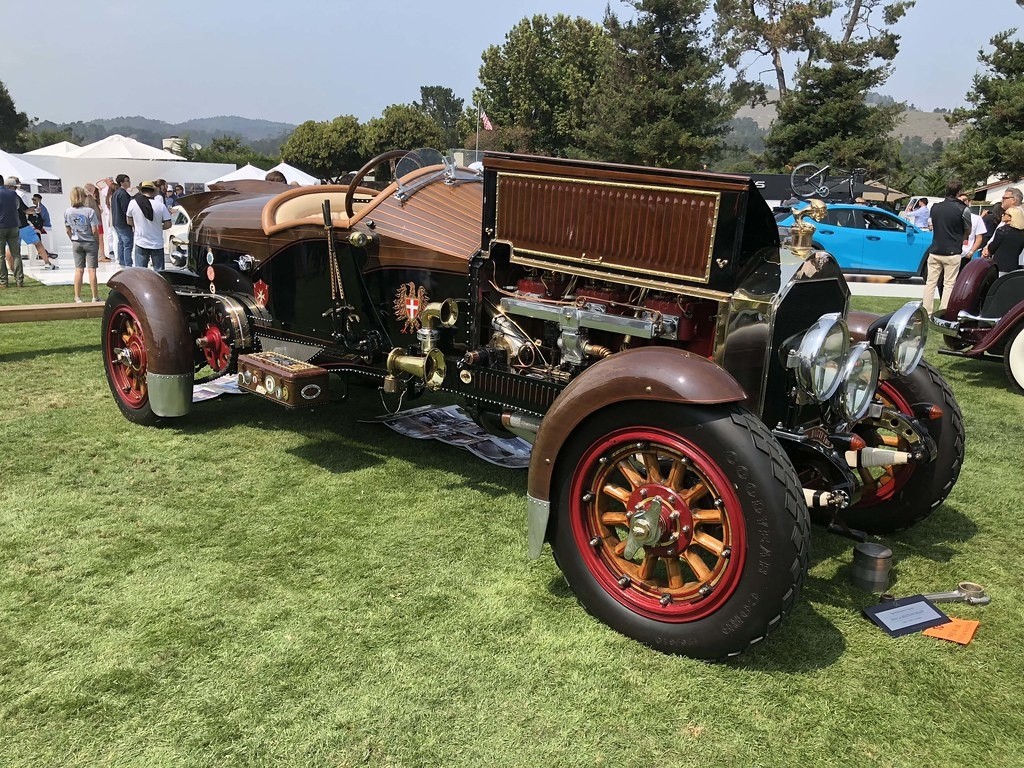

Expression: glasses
xmin=32 ymin=198 xmax=39 ymax=201
xmin=176 ymin=189 xmax=180 ymax=191
xmin=124 ymin=180 xmax=130 ymax=183
xmin=1002 ymin=211 xmax=1011 ymax=217
xmin=12 ymin=186 xmax=17 ymax=190
xmin=1002 ymin=196 xmax=1014 ymax=202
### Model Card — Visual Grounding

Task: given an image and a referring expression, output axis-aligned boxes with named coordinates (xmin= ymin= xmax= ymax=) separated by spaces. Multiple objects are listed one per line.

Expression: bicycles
xmin=791 ymin=149 xmax=869 ymax=203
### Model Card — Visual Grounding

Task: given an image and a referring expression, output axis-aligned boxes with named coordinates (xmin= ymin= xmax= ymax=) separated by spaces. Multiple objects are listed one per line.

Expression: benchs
xmin=261 ymin=185 xmax=378 ymax=225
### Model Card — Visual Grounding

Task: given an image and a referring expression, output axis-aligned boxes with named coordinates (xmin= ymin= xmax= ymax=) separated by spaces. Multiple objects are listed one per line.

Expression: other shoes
xmin=14 ymin=282 xmax=23 ymax=287
xmin=110 ymin=251 xmax=114 ymax=255
xmin=74 ymin=297 xmax=83 ymax=303
xmin=92 ymin=297 xmax=104 ymax=302
xmin=98 ymin=256 xmax=111 ymax=262
xmin=45 ymin=263 xmax=59 ymax=270
xmin=0 ymin=282 xmax=8 ymax=287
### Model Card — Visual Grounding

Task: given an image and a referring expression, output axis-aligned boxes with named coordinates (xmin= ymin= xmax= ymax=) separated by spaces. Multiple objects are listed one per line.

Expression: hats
xmin=136 ymin=181 xmax=156 ymax=190
xmin=4 ymin=178 xmax=21 ymax=186
xmin=166 ymin=184 xmax=173 ymax=191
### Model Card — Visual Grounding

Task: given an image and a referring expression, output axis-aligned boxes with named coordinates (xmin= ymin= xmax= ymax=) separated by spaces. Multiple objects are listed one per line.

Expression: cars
xmin=927 ymin=253 xmax=1024 ymax=397
xmin=100 ymin=148 xmax=965 ymax=665
xmin=161 ymin=204 xmax=192 ymax=268
xmin=772 ymin=200 xmax=980 ymax=284
xmin=898 ymin=196 xmax=945 ymax=231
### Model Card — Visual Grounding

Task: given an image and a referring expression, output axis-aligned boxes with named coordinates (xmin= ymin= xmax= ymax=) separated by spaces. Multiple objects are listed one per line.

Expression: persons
xmin=856 ymin=181 xmax=1024 ymax=322
xmin=0 ymin=169 xmax=305 ymax=302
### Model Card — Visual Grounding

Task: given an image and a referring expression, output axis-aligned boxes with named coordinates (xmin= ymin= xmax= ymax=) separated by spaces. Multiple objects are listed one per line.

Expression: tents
xmin=0 ymin=134 xmax=322 ymax=265
xmin=857 ymin=178 xmax=909 ymax=213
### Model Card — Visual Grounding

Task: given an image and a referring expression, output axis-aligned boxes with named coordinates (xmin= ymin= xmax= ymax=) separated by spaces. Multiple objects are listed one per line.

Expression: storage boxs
xmin=238 ymin=351 xmax=329 ymax=407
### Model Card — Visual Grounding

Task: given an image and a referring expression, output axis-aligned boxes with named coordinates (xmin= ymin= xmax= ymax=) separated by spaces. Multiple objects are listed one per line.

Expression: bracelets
xmin=969 ymin=251 xmax=974 ymax=255
xmin=34 ymin=208 xmax=38 ymax=213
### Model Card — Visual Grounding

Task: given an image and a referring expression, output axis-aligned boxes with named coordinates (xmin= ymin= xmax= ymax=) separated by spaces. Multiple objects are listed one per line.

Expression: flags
xmin=477 ymin=102 xmax=494 ymax=131
